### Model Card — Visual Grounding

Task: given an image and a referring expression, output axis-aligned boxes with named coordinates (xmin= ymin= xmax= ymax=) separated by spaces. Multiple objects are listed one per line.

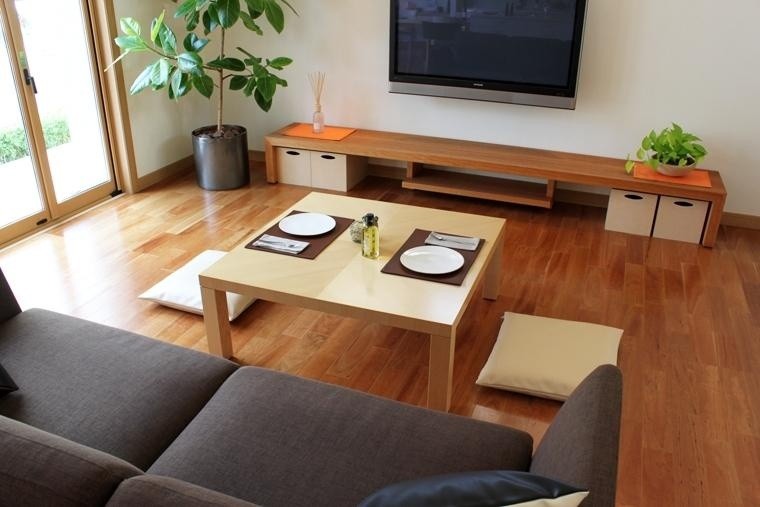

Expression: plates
xmin=399 ymin=246 xmax=465 ymax=276
xmin=278 ymin=213 xmax=337 ymax=237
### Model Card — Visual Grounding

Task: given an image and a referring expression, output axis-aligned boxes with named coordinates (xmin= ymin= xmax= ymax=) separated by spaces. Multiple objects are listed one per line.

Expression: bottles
xmin=362 ymin=213 xmax=379 ymax=260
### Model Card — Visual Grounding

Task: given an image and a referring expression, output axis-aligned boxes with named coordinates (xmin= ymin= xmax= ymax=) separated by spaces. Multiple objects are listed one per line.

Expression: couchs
xmin=0 ymin=268 xmax=623 ymax=507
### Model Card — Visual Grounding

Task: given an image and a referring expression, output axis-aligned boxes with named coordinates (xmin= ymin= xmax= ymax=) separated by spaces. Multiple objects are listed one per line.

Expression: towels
xmin=424 ymin=232 xmax=480 ymax=252
xmin=252 ymin=232 xmax=311 ymax=255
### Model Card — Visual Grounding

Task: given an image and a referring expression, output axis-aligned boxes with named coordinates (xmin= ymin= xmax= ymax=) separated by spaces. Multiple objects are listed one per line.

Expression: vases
xmin=312 ymin=104 xmax=324 ymax=134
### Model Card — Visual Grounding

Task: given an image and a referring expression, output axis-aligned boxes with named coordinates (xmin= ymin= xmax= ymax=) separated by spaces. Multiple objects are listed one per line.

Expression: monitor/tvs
xmin=387 ymin=0 xmax=588 ymax=110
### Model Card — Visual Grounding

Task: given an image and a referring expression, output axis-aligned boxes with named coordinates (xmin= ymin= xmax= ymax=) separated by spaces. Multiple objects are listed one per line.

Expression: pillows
xmin=474 ymin=310 xmax=624 ymax=401
xmin=138 ymin=249 xmax=258 ymax=323
xmin=0 ymin=414 xmax=144 ymax=507
xmin=355 ymin=469 xmax=590 ymax=507
xmin=105 ymin=474 xmax=258 ymax=507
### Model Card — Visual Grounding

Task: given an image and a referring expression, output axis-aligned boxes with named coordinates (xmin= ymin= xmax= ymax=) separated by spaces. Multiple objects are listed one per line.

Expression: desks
xmin=264 ymin=121 xmax=728 ymax=248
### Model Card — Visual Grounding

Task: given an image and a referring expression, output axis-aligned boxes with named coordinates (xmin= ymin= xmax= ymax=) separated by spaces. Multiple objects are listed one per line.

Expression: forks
xmin=428 ymin=230 xmax=478 ymax=246
xmin=256 ymin=238 xmax=303 ymax=250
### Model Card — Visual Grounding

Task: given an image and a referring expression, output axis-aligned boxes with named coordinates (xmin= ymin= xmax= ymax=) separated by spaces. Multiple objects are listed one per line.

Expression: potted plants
xmin=103 ymin=0 xmax=300 ymax=190
xmin=625 ymin=122 xmax=710 ymax=177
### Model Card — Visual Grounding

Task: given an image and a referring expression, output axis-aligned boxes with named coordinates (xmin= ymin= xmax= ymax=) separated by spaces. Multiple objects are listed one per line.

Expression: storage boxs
xmin=311 ymin=147 xmax=369 ymax=193
xmin=604 ymin=189 xmax=659 ymax=237
xmin=652 ymin=194 xmax=710 ymax=244
xmin=276 ymin=146 xmax=311 ymax=187
